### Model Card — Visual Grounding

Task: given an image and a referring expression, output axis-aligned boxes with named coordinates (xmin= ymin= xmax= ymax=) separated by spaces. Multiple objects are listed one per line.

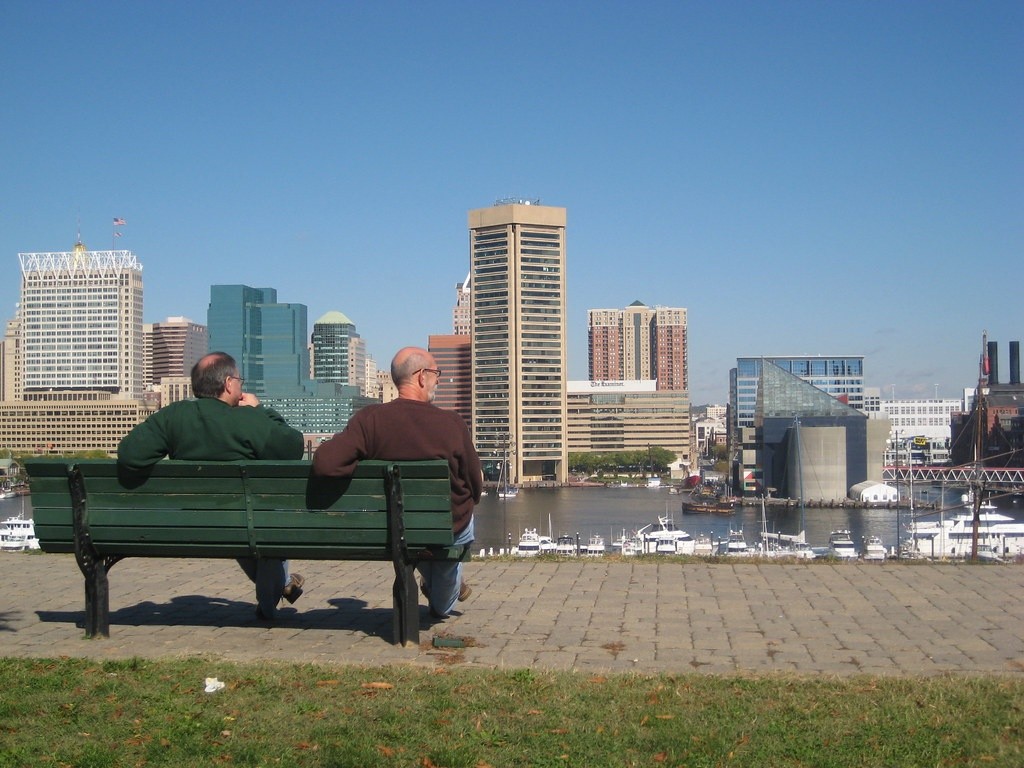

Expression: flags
xmin=115 ymin=233 xmax=121 ymax=236
xmin=114 ymin=218 xmax=126 ymax=226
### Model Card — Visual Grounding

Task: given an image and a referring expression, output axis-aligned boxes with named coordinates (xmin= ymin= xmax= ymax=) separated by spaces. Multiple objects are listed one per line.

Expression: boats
xmin=0 ymin=489 xmax=31 ymax=498
xmin=498 ymin=492 xmax=515 ymax=498
xmin=0 ymin=494 xmax=41 ymax=552
xmin=681 ymin=480 xmax=735 ymax=514
xmin=516 ymin=411 xmax=1024 ymax=562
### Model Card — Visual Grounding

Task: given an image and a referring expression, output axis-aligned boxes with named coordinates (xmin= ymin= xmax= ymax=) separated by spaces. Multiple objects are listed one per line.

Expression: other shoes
xmin=420 ymin=575 xmax=440 ymax=617
xmin=458 ymin=582 xmax=472 ymax=602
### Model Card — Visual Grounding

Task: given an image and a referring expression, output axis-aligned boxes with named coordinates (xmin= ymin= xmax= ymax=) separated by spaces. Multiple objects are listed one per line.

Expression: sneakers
xmin=283 ymin=573 xmax=304 ymax=603
xmin=257 ymin=599 xmax=276 ymax=618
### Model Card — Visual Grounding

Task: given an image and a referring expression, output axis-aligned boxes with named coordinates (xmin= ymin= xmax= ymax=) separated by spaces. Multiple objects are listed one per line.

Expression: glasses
xmin=225 ymin=376 xmax=245 ymax=386
xmin=412 ymin=368 xmax=442 ymax=378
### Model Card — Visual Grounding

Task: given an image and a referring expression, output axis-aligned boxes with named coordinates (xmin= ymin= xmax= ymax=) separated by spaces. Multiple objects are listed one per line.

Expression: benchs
xmin=23 ymin=458 xmax=472 ymax=648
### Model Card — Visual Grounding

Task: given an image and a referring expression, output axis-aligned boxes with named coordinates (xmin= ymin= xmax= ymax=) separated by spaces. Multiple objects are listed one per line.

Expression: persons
xmin=118 ymin=352 xmax=305 ymax=618
xmin=313 ymin=347 xmax=482 ymax=616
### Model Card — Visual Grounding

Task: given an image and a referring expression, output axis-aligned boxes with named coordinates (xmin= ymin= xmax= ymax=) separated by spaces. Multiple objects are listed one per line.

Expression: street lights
xmin=886 ymin=427 xmax=906 ymax=562
xmin=492 ymin=433 xmax=516 ymax=555
